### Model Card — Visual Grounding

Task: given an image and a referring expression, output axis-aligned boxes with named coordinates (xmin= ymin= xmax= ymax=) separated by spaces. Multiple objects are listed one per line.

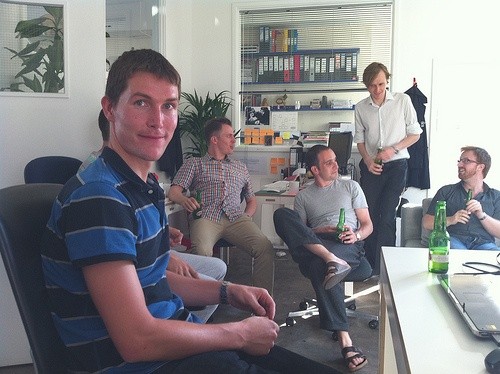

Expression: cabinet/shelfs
xmin=241 ymin=48 xmax=360 ymax=113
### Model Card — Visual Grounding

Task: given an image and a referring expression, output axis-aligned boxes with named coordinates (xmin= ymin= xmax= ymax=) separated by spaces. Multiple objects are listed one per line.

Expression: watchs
xmin=355 ymin=232 xmax=361 ymax=241
xmin=392 ymin=145 xmax=399 ymax=154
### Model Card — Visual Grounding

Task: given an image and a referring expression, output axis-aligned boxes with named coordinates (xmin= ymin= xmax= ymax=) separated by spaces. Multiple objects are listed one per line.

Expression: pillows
xmin=421 ymin=199 xmax=432 ymax=246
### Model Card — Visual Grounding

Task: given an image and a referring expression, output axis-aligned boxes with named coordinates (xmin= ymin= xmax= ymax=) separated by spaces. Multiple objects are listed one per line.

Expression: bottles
xmin=192 ymin=190 xmax=203 ymax=220
xmin=295 ymin=101 xmax=300 ymax=110
xmin=374 ymin=147 xmax=382 ymax=170
xmin=428 ymin=200 xmax=450 ymax=273
xmin=464 ymin=189 xmax=472 ymax=215
xmin=336 ymin=208 xmax=347 ymax=243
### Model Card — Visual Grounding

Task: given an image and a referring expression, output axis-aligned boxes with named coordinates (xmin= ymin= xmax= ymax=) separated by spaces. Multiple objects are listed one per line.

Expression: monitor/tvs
xmin=327 ymin=130 xmax=353 ymax=173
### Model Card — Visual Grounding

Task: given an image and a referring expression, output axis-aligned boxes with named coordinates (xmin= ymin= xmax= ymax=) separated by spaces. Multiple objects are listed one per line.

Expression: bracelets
xmin=479 ymin=215 xmax=487 ymax=220
xmin=220 ymin=281 xmax=229 ymax=304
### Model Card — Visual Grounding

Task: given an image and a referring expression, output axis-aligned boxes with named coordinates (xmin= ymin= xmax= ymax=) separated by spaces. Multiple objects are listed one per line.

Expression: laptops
xmin=442 ymin=275 xmax=500 ymax=339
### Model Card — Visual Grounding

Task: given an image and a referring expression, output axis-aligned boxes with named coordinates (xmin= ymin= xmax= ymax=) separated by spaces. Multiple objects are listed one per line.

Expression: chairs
xmin=0 ymin=132 xmax=432 ymax=374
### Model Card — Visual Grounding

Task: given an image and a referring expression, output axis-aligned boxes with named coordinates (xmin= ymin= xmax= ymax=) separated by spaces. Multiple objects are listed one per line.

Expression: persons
xmin=40 ymin=49 xmax=337 ymax=374
xmin=246 ymin=107 xmax=269 ymax=125
xmin=273 ymin=145 xmax=373 ymax=371
xmin=168 ymin=116 xmax=274 ymax=292
xmin=354 ymin=62 xmax=423 ymax=273
xmin=422 ymin=146 xmax=500 ymax=250
xmin=76 ymin=110 xmax=227 ymax=322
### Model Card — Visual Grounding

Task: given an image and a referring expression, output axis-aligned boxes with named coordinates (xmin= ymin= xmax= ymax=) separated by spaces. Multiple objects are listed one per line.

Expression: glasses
xmin=458 ymin=159 xmax=479 ymax=164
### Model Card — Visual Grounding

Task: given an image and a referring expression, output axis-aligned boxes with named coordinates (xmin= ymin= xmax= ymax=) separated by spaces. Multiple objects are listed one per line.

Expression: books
xmin=304 ymin=122 xmax=351 ymax=143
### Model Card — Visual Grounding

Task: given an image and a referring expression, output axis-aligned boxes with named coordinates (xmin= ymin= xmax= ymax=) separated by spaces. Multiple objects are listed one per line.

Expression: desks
xmin=165 ymin=191 xmax=187 ymax=215
xmin=378 ymin=246 xmax=500 ymax=374
xmin=252 ymin=190 xmax=297 ymax=249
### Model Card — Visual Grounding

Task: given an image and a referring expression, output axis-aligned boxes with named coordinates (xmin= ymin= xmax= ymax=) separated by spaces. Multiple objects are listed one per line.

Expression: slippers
xmin=322 ymin=262 xmax=351 ymax=290
xmin=342 ymin=346 xmax=368 ymax=372
